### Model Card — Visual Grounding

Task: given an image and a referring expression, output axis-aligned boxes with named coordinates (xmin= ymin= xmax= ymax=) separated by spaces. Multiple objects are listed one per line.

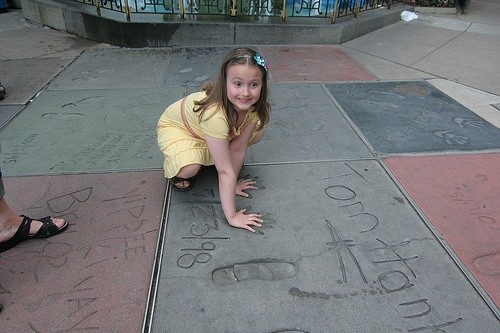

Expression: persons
xmin=156 ymin=46 xmax=273 ymax=232
xmin=1 ymin=171 xmax=69 ymax=312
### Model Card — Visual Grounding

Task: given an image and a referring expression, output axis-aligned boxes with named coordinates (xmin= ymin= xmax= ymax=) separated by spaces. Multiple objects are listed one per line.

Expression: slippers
xmin=0 ymin=215 xmax=69 ymax=252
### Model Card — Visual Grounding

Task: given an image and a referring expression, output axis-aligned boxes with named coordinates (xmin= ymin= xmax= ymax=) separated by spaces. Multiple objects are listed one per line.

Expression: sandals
xmin=171 ymin=176 xmax=192 ymax=191
